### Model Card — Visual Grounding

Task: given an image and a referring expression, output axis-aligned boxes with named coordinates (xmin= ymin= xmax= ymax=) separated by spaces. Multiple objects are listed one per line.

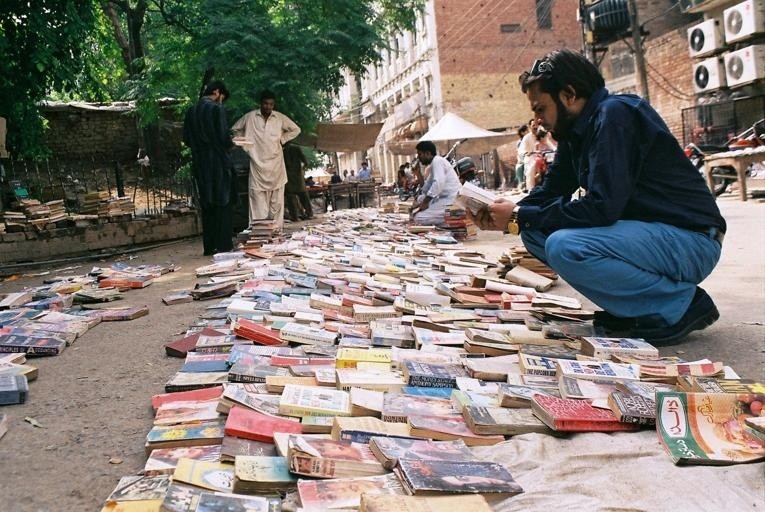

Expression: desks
xmin=310 ymin=180 xmax=381 ymax=212
xmin=701 ymin=146 xmax=765 ymax=201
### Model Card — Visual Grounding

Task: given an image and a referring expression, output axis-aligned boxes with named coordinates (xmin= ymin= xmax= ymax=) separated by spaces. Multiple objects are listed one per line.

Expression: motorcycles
xmin=528 ymin=149 xmax=556 ymax=178
xmin=305 ymin=184 xmax=327 ymax=211
xmin=444 ymin=139 xmax=485 ymax=189
xmin=392 ymin=181 xmax=423 ymax=204
xmin=680 ymin=117 xmax=765 ymax=197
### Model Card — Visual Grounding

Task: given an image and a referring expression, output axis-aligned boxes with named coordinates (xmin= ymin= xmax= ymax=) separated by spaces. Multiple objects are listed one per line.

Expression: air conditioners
xmin=722 ymin=0 xmax=765 ymax=45
xmin=687 ymin=18 xmax=726 ymax=58
xmin=692 ymin=56 xmax=726 ymax=96
xmin=725 ymin=44 xmax=765 ymax=89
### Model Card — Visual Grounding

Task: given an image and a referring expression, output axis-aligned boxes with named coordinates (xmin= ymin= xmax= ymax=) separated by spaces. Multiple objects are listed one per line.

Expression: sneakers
xmin=592 ymin=299 xmax=634 ymax=339
xmin=633 ymin=299 xmax=723 ymax=349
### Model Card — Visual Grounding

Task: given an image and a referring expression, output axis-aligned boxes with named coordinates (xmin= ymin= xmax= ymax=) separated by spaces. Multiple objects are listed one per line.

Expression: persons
xmin=515 ymin=119 xmax=558 ymax=195
xmin=229 ymin=91 xmax=301 ymax=228
xmin=465 ymin=50 xmax=727 ymax=346
xmin=184 ymin=81 xmax=238 ymax=257
xmin=283 ymin=142 xmax=313 ymax=221
xmin=410 ymin=141 xmax=463 ymax=225
xmin=305 ymin=155 xmax=423 ymax=197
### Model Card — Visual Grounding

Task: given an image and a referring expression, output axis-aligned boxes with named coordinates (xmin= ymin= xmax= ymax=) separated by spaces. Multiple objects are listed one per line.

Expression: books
xmin=0 ymin=191 xmax=154 ymax=406
xmin=102 ymin=207 xmax=765 ymax=512
